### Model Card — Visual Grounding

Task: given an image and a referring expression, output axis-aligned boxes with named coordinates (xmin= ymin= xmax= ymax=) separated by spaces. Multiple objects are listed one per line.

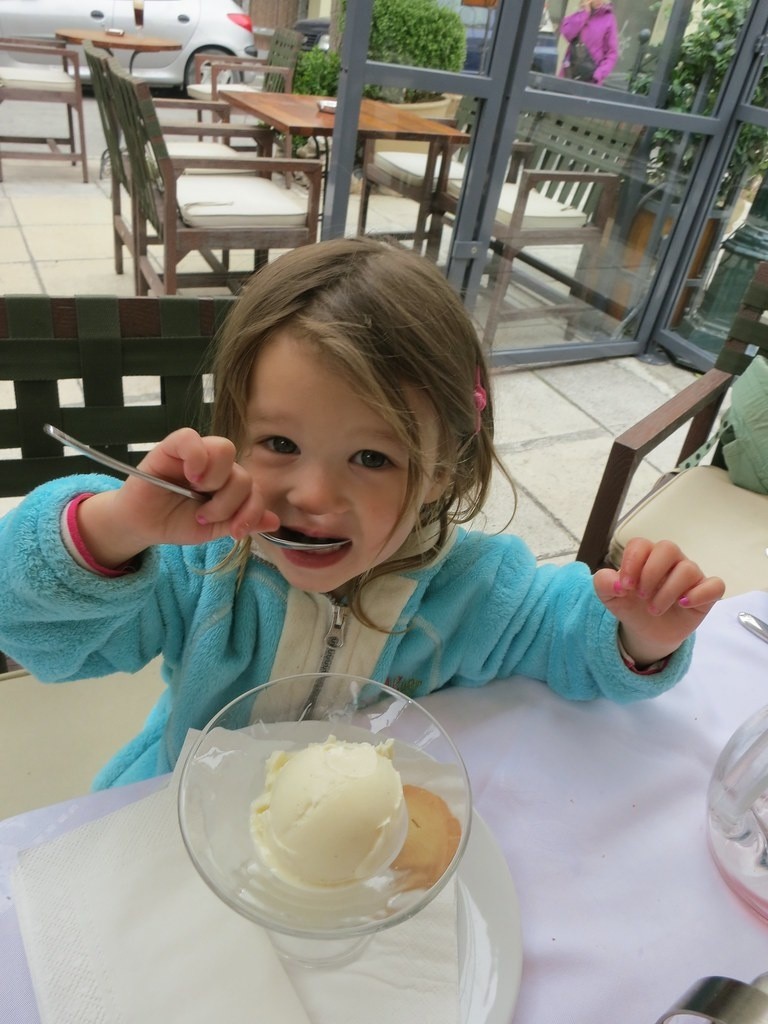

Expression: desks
xmin=1 ymin=590 xmax=768 ymax=1024
xmin=221 ymin=90 xmax=470 ymax=257
xmin=54 ymin=29 xmax=183 ymax=181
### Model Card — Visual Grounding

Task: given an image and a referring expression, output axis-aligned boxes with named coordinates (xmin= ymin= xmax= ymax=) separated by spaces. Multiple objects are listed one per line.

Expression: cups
xmin=176 ymin=674 xmax=473 ymax=971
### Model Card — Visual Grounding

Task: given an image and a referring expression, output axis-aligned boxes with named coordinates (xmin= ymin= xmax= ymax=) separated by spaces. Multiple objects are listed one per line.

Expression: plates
xmin=268 ymin=808 xmax=523 ymax=1024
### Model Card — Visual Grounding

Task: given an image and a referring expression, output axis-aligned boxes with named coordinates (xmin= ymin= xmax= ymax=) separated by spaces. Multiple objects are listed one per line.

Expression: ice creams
xmin=246 ymin=737 xmax=409 ymax=889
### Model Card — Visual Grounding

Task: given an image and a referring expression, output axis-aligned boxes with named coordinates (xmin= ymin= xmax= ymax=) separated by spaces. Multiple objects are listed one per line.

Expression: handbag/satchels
xmin=570 ymin=41 xmax=597 ymax=84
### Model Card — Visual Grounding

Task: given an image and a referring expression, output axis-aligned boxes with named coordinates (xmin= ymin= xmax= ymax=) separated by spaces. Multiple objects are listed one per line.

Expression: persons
xmin=559 ymin=0 xmax=619 ymax=84
xmin=0 ymin=236 xmax=725 ymax=796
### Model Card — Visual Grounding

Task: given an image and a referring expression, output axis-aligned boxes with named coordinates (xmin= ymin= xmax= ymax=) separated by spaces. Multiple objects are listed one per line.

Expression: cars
xmin=0 ymin=0 xmax=258 ymax=101
xmin=292 ymin=0 xmax=557 ymax=91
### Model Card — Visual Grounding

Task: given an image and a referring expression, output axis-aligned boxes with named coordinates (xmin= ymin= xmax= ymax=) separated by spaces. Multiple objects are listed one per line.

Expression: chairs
xmin=1 ymin=31 xmax=86 ymax=184
xmin=102 ymin=57 xmax=326 ymax=300
xmin=573 ymin=261 xmax=768 ymax=598
xmin=426 ymin=107 xmax=648 ymax=366
xmin=0 ymin=299 xmax=242 ymax=676
xmin=85 ymin=49 xmax=274 ymax=295
xmin=192 ymin=28 xmax=306 ymax=189
xmin=357 ymin=94 xmax=541 ymax=258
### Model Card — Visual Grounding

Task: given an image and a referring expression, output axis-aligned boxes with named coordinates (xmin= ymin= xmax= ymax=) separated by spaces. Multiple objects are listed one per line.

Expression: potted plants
xmin=566 ymin=1 xmax=768 ymax=335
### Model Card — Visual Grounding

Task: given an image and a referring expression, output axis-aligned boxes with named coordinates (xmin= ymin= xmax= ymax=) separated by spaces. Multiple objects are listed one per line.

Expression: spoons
xmin=44 ymin=424 xmax=351 ymax=553
